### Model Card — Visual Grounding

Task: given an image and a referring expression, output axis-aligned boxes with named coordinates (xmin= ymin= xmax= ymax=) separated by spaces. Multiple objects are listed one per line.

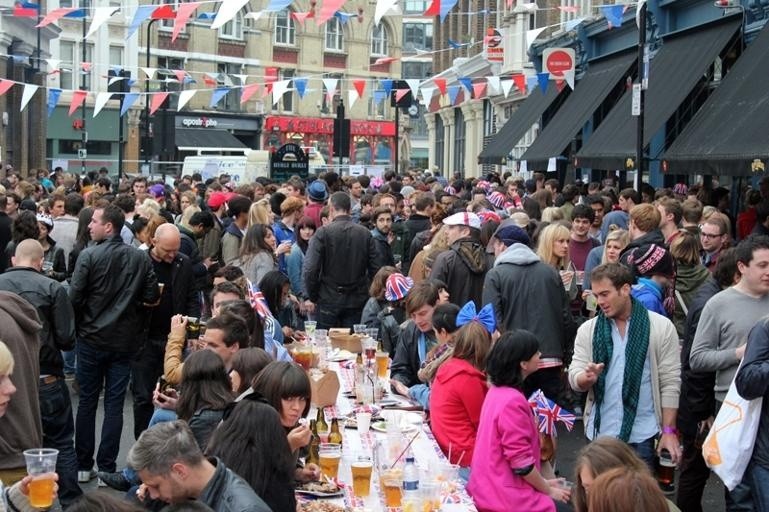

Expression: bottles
xmin=305 ymin=407 xmax=343 ymax=464
xmin=354 ymin=353 xmax=364 ymax=388
xmin=181 ymin=316 xmax=207 ymax=332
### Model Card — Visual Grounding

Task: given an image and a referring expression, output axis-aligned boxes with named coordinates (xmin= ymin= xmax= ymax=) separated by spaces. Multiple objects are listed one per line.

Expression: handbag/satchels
xmin=701 ymin=354 xmax=764 ymax=490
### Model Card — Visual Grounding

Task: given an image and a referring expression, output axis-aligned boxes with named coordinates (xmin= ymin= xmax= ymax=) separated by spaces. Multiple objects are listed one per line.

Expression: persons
xmin=0 ymin=161 xmax=415 ymax=512
xmin=404 ymin=162 xmax=768 ymax=512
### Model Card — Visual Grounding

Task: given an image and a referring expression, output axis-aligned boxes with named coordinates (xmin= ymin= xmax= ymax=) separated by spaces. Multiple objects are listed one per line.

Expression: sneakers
xmin=79 ymin=467 xmax=99 ymax=482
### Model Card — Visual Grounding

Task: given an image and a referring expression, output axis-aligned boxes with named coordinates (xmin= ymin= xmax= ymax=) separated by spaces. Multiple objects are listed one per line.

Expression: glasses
xmin=699 ymin=232 xmax=723 ymax=240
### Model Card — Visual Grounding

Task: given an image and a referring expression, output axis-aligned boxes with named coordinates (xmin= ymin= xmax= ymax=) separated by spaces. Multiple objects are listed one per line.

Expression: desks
xmin=291 ymin=330 xmax=478 ymax=511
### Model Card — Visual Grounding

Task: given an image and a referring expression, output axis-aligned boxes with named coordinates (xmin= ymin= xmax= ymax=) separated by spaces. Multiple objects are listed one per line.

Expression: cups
xmin=42 ymin=261 xmax=53 ymax=276
xmin=553 ymin=480 xmax=573 ymax=493
xmin=23 ymin=448 xmax=59 ymax=508
xmin=290 ymin=320 xmax=460 ymax=511
xmin=660 ymin=453 xmax=675 ymax=487
xmin=281 ymin=240 xmax=293 ymax=255
xmin=559 ymin=270 xmax=597 ymax=312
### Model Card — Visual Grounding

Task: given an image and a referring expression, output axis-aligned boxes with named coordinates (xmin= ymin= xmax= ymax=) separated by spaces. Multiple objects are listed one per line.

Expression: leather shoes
xmin=100 ymin=471 xmax=135 ymax=492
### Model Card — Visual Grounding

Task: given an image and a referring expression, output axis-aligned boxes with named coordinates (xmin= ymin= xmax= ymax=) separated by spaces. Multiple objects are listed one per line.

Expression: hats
xmin=370 ymin=177 xmax=385 ymax=190
xmin=34 ymin=212 xmax=53 ymax=231
xmin=628 ymin=244 xmax=675 ymax=279
xmin=147 ymin=184 xmax=166 ymax=204
xmin=673 ymin=183 xmax=687 ymax=196
xmin=384 ymin=272 xmax=415 ymax=302
xmin=208 ymin=193 xmax=236 ymax=209
xmin=306 ymin=182 xmax=329 ymax=202
xmin=399 ymin=166 xmax=530 ymax=246
